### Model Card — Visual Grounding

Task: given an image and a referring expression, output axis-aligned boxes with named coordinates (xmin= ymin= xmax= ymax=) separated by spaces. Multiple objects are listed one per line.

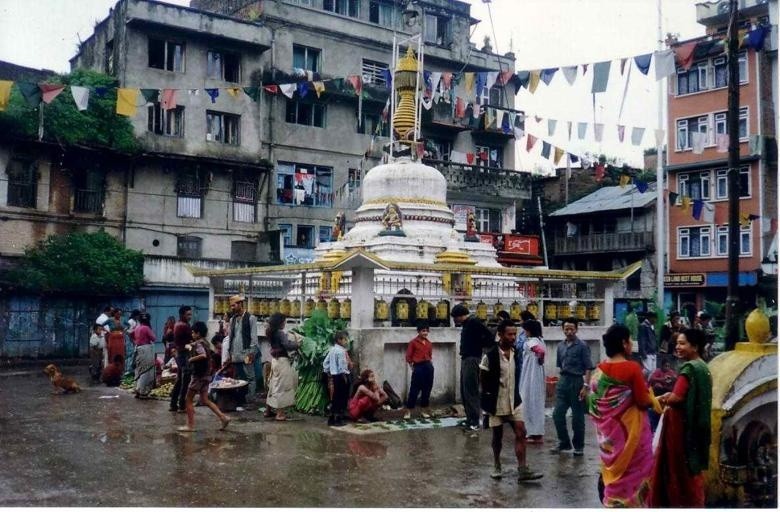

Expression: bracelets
xmin=583 ymin=382 xmax=589 ymax=388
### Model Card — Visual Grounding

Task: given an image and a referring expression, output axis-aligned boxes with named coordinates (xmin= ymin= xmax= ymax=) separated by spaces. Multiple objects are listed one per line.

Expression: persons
xmin=550 ymin=318 xmax=596 ymax=455
xmin=323 ymin=330 xmax=389 ymax=426
xmin=403 ymin=325 xmax=434 ymax=420
xmin=587 ymin=324 xmax=667 ymax=507
xmin=641 ymin=310 xmax=712 ymax=430
xmin=660 ymin=329 xmax=713 ymax=508
xmin=89 ymin=305 xmax=303 ymax=433
xmin=453 ymin=305 xmax=548 ymax=483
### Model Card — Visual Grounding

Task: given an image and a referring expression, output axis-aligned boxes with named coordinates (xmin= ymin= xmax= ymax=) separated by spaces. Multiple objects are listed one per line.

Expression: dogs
xmin=43 ymin=364 xmax=81 ymax=394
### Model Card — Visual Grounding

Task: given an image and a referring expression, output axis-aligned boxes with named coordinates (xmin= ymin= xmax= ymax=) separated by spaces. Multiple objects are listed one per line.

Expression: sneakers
xmin=460 ymin=420 xmax=480 ymax=433
xmin=550 ymin=440 xmax=584 ymax=456
xmin=236 ymin=407 xmax=245 ymax=411
xmin=518 ymin=472 xmax=544 ymax=481
xmin=404 ymin=409 xmax=431 ymax=419
xmin=526 ymin=435 xmax=544 ymax=445
xmin=490 ymin=469 xmax=502 ymax=479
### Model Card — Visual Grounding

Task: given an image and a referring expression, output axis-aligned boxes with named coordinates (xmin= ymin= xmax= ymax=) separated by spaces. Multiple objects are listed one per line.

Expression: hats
xmin=229 ymin=294 xmax=241 ymax=305
